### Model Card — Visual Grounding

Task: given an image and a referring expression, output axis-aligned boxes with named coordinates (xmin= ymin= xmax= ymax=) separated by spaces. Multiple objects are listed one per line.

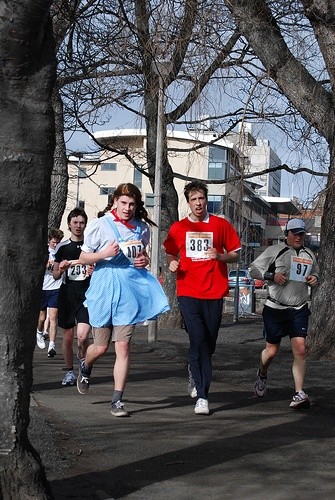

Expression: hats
xmin=286 ymin=218 xmax=309 ymax=235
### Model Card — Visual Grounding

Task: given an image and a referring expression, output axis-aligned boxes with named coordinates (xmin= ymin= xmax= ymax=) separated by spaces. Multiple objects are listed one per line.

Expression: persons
xmin=37 ymin=229 xmax=64 ymax=357
xmin=77 ymin=183 xmax=171 ymax=416
xmin=54 ymin=209 xmax=94 ymax=386
xmin=164 ymin=182 xmax=242 ymax=413
xmin=250 ymin=220 xmax=320 ymax=408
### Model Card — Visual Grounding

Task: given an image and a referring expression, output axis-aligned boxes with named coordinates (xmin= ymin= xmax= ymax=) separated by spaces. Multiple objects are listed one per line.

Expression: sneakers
xmin=187 ymin=364 xmax=198 ymax=398
xmin=253 ymin=369 xmax=267 ymax=397
xmin=77 ymin=352 xmax=84 ymax=363
xmin=194 ymin=398 xmax=209 ymax=414
xmin=290 ymin=390 xmax=310 ymax=407
xmin=47 ymin=346 xmax=56 ymax=358
xmin=110 ymin=400 xmax=128 ymax=416
xmin=62 ymin=370 xmax=76 ymax=385
xmin=37 ymin=333 xmax=46 ymax=349
xmin=76 ymin=358 xmax=92 ymax=394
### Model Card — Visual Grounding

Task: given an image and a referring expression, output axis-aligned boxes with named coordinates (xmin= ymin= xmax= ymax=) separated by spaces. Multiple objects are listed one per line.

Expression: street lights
xmin=69 ymin=151 xmax=84 ymax=209
xmin=233 ymin=153 xmax=249 ymax=324
xmin=145 ymin=52 xmax=174 ymax=345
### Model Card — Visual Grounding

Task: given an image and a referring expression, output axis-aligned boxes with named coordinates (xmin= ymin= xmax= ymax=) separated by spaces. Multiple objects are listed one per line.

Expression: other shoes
xmin=43 ymin=331 xmax=49 ymax=339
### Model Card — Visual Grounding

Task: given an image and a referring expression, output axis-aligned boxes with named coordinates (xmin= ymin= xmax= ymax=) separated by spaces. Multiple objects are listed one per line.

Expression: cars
xmin=228 ymin=268 xmax=266 ymax=290
